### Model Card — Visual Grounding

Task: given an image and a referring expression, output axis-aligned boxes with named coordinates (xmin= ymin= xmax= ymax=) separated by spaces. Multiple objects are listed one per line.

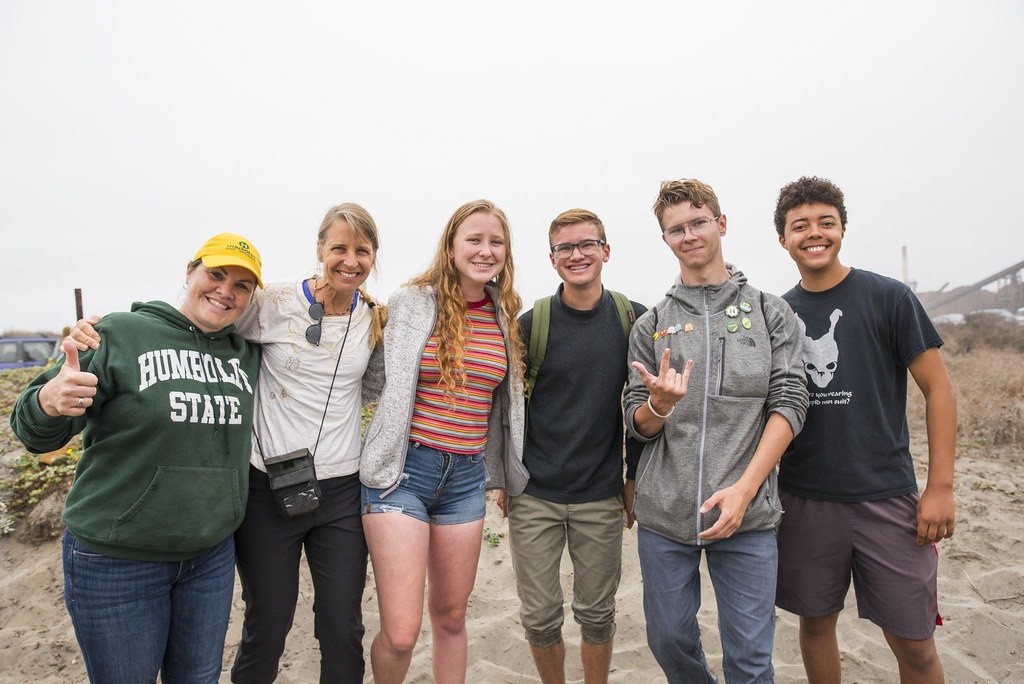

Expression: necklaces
xmin=314 ymin=277 xmax=355 ymax=315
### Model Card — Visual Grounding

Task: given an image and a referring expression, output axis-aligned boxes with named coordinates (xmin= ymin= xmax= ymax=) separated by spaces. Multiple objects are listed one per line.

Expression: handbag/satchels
xmin=264 ymin=448 xmax=322 ymax=518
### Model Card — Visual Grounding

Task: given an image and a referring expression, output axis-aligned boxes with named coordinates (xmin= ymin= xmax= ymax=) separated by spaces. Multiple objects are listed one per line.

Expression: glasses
xmin=661 ymin=216 xmax=719 ymax=243
xmin=550 ymin=239 xmax=606 ymax=259
xmin=306 ymin=300 xmax=327 ymax=346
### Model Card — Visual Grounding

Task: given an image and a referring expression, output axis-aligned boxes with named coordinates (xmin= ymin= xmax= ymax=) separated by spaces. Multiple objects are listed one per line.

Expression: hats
xmin=191 ymin=233 xmax=264 ymax=291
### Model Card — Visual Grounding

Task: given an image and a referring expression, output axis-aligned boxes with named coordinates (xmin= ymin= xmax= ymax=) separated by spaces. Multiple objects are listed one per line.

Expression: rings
xmin=77 ymin=397 xmax=84 ymax=408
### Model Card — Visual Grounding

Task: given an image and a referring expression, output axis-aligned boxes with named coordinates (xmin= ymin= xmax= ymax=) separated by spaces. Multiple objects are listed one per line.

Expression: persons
xmin=494 ymin=209 xmax=648 ymax=684
xmin=60 ymin=202 xmax=389 ymax=684
xmin=771 ymin=176 xmax=958 ymax=684
xmin=10 ymin=232 xmax=263 ymax=684
xmin=359 ymin=199 xmax=531 ymax=684
xmin=623 ymin=177 xmax=809 ymax=684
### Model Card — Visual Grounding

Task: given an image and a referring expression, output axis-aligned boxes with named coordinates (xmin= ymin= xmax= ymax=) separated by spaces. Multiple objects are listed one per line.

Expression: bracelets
xmin=648 ymin=395 xmax=674 ymax=418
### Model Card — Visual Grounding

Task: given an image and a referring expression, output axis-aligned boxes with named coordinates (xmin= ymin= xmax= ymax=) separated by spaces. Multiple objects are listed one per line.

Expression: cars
xmin=0 ymin=337 xmax=62 ymax=370
